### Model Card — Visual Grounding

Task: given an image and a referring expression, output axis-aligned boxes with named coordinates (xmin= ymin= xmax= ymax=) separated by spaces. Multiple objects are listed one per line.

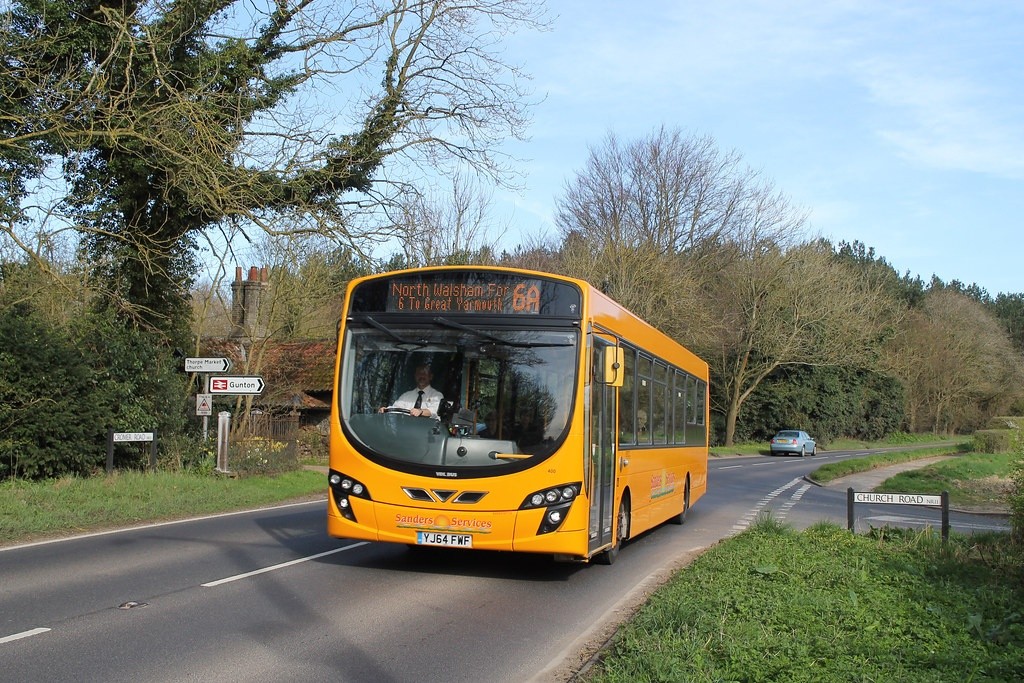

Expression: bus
xmin=327 ymin=266 xmax=710 ymax=567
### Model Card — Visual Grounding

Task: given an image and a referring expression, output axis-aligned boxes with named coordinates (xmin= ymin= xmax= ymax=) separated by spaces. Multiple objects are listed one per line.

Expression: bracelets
xmin=419 ymin=411 xmax=423 ymax=416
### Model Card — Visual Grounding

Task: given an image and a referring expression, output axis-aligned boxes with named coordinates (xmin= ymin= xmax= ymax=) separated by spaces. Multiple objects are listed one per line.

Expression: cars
xmin=770 ymin=429 xmax=817 ymax=457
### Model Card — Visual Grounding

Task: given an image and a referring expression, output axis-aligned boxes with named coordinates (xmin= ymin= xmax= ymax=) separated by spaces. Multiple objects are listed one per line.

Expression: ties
xmin=414 ymin=391 xmax=424 ymax=408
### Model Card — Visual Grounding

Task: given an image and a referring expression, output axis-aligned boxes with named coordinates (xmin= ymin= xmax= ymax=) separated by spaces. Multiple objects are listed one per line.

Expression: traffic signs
xmin=207 ymin=375 xmax=266 ymax=396
xmin=185 ymin=357 xmax=233 ymax=373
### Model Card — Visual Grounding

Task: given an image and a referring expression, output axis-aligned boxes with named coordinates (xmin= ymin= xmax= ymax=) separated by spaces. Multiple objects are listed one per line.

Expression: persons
xmin=377 ymin=364 xmax=444 ymax=422
xmin=637 ymin=410 xmax=648 ymax=433
xmin=476 ymin=408 xmax=539 ymax=449
